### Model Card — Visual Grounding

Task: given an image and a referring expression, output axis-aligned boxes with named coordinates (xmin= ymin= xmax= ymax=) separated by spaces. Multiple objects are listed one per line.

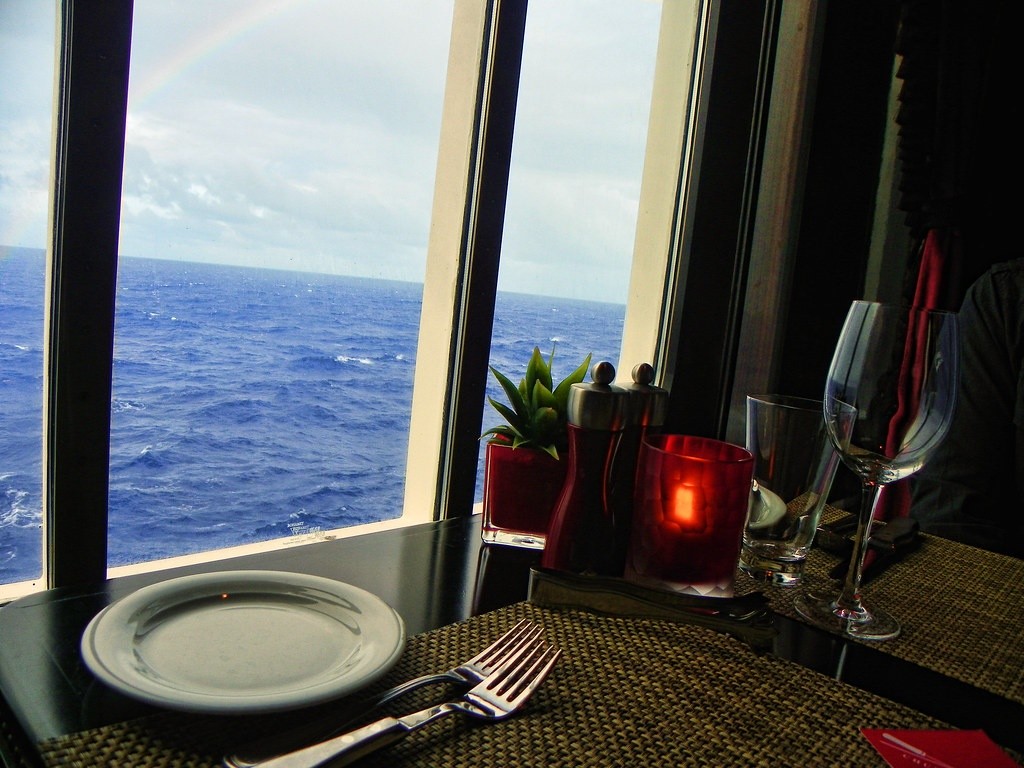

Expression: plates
xmin=79 ymin=570 xmax=406 ymax=711
xmin=746 ymin=485 xmax=786 ymax=528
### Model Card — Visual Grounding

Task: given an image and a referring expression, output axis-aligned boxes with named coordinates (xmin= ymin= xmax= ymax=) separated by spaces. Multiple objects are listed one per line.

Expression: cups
xmin=736 ymin=394 xmax=857 ymax=587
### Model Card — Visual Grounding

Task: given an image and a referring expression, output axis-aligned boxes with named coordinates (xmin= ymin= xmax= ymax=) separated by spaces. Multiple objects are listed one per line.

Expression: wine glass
xmin=792 ymin=301 xmax=958 ymax=640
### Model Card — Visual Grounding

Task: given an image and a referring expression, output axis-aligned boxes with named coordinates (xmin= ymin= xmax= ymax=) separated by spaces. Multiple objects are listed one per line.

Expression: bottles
xmin=617 ymin=363 xmax=672 ymax=578
xmin=543 ymin=362 xmax=632 ymax=580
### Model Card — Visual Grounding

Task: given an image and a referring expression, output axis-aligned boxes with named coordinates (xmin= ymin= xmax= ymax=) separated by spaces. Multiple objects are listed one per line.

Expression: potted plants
xmin=482 ymin=344 xmax=593 ymax=549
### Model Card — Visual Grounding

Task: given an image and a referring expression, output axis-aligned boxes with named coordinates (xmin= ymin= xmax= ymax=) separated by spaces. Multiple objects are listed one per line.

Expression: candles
xmin=633 ymin=428 xmax=755 ymax=577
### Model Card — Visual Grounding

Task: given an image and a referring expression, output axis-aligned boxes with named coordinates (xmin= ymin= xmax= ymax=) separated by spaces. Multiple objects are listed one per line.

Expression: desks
xmin=0 ymin=498 xmax=1024 ymax=768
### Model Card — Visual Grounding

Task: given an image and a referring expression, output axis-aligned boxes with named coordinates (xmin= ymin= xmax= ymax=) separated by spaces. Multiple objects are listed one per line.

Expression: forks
xmin=221 ymin=617 xmax=544 ymax=768
xmin=255 ymin=642 xmax=563 ymax=768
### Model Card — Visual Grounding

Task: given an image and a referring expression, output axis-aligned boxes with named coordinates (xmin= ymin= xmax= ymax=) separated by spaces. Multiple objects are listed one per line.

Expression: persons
xmin=903 ymin=258 xmax=1024 ymax=560
